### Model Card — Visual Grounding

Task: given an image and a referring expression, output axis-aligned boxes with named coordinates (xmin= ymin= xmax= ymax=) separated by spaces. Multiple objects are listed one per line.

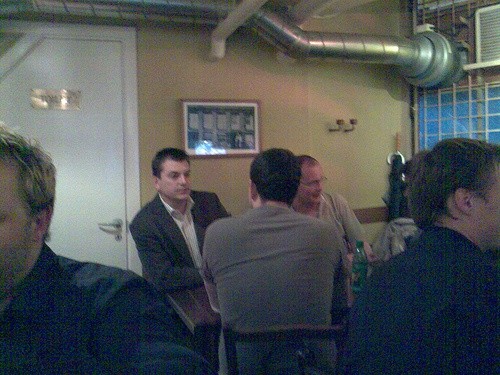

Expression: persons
xmin=341 ymin=138 xmax=500 ymax=375
xmin=0 ymin=119 xmax=214 ymax=375
xmin=292 ymin=154 xmax=376 ymax=269
xmin=201 ymin=147 xmax=349 ymax=375
xmin=128 ymin=146 xmax=232 ymax=298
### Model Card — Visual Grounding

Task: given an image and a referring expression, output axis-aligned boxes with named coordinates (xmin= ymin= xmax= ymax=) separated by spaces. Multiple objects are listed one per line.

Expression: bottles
xmin=351 ymin=240 xmax=368 ymax=296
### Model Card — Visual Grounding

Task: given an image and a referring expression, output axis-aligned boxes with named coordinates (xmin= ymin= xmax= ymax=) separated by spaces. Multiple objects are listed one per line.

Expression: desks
xmin=341 ymin=250 xmax=386 ymax=307
xmin=167 ymin=285 xmax=220 ymax=337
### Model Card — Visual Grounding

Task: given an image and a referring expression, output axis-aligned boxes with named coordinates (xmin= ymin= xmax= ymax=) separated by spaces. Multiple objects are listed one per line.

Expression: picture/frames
xmin=176 ymin=98 xmax=264 ymax=159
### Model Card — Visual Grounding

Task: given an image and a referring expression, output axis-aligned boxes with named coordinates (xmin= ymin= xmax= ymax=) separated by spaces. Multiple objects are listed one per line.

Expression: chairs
xmin=220 ymin=318 xmax=345 ymax=375
